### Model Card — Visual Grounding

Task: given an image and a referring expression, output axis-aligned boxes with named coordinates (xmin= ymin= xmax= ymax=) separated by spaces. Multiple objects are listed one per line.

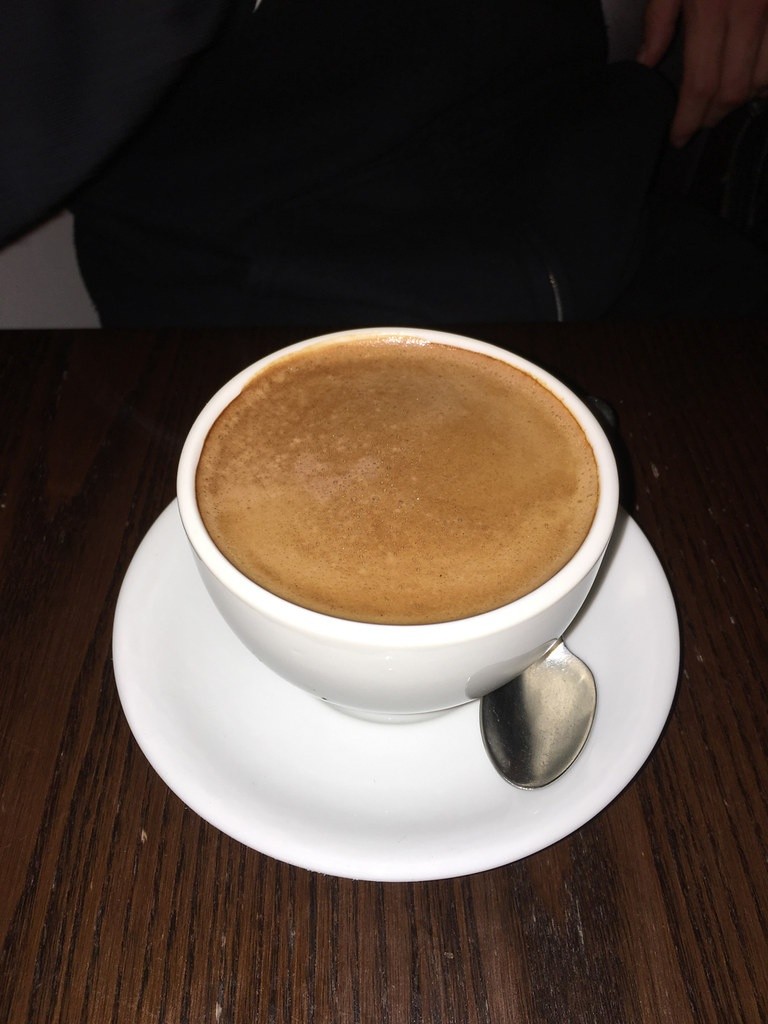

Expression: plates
xmin=113 ymin=497 xmax=680 ymax=885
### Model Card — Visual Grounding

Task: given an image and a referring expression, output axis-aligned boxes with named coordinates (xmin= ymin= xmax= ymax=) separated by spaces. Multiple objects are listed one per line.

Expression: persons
xmin=0 ymin=0 xmax=768 ymax=327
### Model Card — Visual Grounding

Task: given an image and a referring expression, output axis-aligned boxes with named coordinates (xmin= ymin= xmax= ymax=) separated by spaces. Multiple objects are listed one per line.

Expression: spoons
xmin=479 ymin=397 xmax=618 ymax=791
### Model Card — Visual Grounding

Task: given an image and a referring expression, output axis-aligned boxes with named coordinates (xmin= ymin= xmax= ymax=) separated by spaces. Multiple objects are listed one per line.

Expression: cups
xmin=176 ymin=327 xmax=623 ymax=723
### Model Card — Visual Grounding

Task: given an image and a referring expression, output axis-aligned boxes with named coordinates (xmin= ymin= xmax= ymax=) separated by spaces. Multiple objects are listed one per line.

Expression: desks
xmin=1 ymin=317 xmax=767 ymax=1024
